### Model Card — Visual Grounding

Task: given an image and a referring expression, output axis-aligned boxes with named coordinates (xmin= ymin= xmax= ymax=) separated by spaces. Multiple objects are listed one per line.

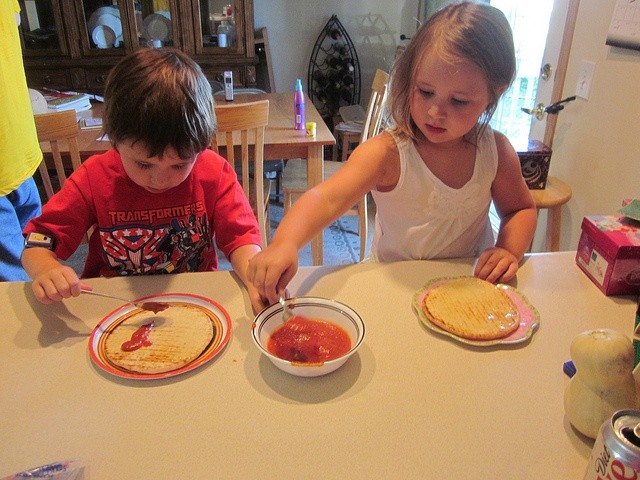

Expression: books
xmin=46 ymin=94 xmax=91 ymax=114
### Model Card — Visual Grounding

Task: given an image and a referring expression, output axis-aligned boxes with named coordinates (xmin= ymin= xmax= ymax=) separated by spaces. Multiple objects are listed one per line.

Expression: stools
xmin=529 ymin=177 xmax=571 ymax=253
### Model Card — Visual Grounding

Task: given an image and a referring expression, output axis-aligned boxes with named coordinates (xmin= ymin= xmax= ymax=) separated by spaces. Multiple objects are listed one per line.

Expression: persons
xmin=1 ymin=0 xmax=44 ymax=282
xmin=245 ymin=3 xmax=538 ymax=306
xmin=21 ymin=47 xmax=291 ymax=315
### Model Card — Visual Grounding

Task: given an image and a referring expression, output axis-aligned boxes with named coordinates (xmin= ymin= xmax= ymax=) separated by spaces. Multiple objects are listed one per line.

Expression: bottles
xmin=216 ymin=25 xmax=230 ymax=48
xmin=305 ymin=122 xmax=317 ymax=136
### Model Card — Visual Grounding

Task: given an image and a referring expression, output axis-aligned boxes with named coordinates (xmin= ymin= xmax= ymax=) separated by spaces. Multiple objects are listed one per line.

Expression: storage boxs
xmin=512 ymin=139 xmax=553 ymax=189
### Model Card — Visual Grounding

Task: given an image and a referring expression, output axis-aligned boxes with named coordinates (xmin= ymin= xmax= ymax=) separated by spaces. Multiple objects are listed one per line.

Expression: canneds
xmin=582 ymin=408 xmax=640 ymax=480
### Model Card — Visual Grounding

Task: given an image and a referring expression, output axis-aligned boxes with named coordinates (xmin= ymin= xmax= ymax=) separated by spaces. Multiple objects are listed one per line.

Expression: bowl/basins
xmin=251 ymin=297 xmax=365 ymax=377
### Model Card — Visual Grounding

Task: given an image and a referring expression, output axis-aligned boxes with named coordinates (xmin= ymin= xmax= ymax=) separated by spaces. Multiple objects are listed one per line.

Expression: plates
xmin=91 ymin=13 xmax=124 ymax=49
xmin=141 ymin=14 xmax=173 ymax=45
xmin=412 ymin=276 xmax=540 ymax=346
xmin=88 ymin=6 xmax=121 ymax=36
xmin=88 ymin=294 xmax=232 ymax=379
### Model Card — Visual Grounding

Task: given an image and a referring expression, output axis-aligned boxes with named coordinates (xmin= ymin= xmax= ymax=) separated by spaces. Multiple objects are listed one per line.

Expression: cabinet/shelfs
xmin=307 ymin=13 xmax=361 ymax=151
xmin=17 ymin=0 xmax=259 ymax=96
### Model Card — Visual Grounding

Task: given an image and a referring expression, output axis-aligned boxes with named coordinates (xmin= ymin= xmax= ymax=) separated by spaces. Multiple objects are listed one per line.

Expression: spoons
xmin=80 ymin=289 xmax=169 ymax=311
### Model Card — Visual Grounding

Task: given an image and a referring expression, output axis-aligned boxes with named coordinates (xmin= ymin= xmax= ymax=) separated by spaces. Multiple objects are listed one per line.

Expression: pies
xmin=105 ymin=305 xmax=213 ymax=373
xmin=424 ymin=277 xmax=521 ymax=340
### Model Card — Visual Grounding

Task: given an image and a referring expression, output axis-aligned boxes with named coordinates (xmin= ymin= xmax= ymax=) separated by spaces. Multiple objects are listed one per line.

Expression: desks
xmin=33 ymin=92 xmax=336 ymax=265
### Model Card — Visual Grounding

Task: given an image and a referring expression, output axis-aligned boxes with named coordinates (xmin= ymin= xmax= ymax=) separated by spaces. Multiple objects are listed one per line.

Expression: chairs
xmin=210 ymin=100 xmax=269 ymax=247
xmin=332 ymin=45 xmax=407 ymax=162
xmin=280 ymin=68 xmax=393 ymax=265
xmin=34 ymin=109 xmax=94 ymax=242
